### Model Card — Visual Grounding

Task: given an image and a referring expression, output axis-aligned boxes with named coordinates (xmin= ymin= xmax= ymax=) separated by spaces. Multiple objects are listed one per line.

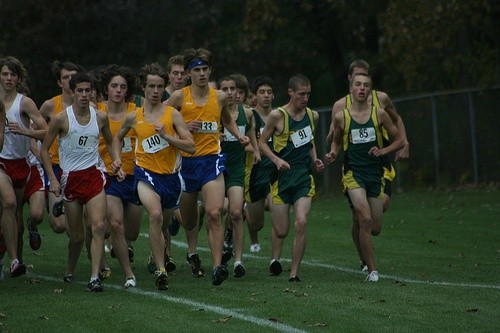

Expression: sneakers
xmin=165 ymin=257 xmax=176 ymax=272
xmin=168 ymin=214 xmax=179 ymax=236
xmin=89 ymin=280 xmax=103 ymax=292
xmin=0 ymin=263 xmax=4 ymax=281
xmin=127 ymin=246 xmax=134 ymax=262
xmin=27 ymin=218 xmax=41 ymax=250
xmin=232 ymin=261 xmax=246 ymax=278
xmin=212 ymin=266 xmax=228 ymax=287
xmin=100 ymin=266 xmax=111 ymax=278
xmin=147 ymin=251 xmax=157 ymax=273
xmin=249 ymin=243 xmax=260 ymax=253
xmin=186 ymin=253 xmax=206 ymax=277
xmin=63 ymin=274 xmax=73 ymax=285
xmin=52 ymin=197 xmax=63 ymax=217
xmin=154 ymin=270 xmax=168 ymax=290
xmin=270 ymin=258 xmax=282 ymax=276
xmin=221 ymin=252 xmax=231 ymax=265
xmin=361 ymin=261 xmax=367 ymax=271
xmin=223 ymin=229 xmax=234 ymax=252
xmin=289 ymin=276 xmax=300 ymax=282
xmin=125 ymin=276 xmax=136 ymax=287
xmin=369 ymin=271 xmax=379 ymax=282
xmin=10 ymin=259 xmax=26 ymax=278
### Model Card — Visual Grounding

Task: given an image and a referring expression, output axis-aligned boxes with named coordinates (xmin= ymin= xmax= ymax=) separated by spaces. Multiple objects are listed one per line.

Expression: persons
xmin=325 ymin=58 xmax=409 ymax=271
xmin=245 ymin=78 xmax=274 ymax=253
xmin=259 ymin=73 xmax=324 ymax=282
xmin=0 ymin=55 xmax=125 ymax=292
xmin=325 ymin=71 xmax=405 ymax=281
xmin=99 ymin=48 xmax=261 ymax=292
